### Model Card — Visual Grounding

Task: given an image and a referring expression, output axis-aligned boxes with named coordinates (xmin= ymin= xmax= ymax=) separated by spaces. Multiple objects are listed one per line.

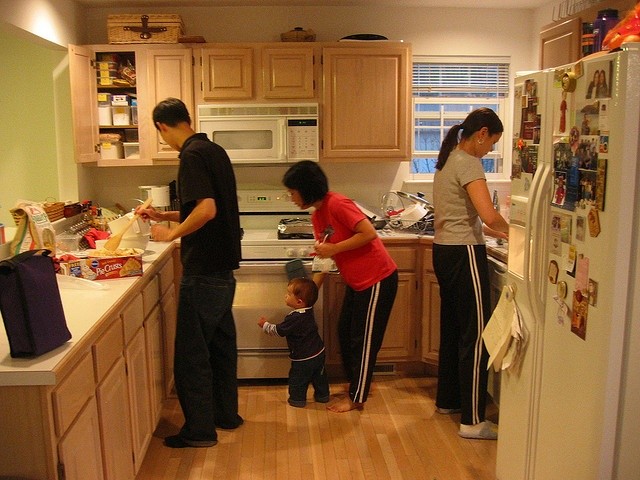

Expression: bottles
xmin=593 ymin=8 xmax=621 ymax=53
xmin=94 ymin=208 xmax=106 ymax=231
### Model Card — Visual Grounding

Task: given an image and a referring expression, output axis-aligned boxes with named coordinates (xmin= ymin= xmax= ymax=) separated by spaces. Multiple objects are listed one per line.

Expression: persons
xmin=257 ymin=278 xmax=330 ymax=408
xmin=433 ymin=107 xmax=510 ymax=439
xmin=282 ymin=160 xmax=399 ymax=412
xmin=134 ymin=98 xmax=244 ymax=447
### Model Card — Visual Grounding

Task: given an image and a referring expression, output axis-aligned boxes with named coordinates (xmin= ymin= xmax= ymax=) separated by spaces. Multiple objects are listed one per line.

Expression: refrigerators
xmin=494 ymin=49 xmax=639 ymax=476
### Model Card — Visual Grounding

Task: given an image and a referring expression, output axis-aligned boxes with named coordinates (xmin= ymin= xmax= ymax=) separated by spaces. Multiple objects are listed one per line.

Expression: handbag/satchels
xmin=0 ymin=247 xmax=71 ymax=357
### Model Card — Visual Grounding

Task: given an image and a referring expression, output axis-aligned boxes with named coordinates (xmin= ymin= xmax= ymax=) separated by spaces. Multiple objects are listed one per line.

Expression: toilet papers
xmin=151 ymin=186 xmax=171 ymax=207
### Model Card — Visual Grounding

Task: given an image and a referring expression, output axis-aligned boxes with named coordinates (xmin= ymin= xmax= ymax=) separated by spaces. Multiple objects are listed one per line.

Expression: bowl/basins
xmin=95 ymin=234 xmax=151 ymax=254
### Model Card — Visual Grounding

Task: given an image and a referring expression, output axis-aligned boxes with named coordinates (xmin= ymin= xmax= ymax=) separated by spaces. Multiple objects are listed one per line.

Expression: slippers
xmin=459 ymin=421 xmax=499 ymax=440
xmin=434 ymin=406 xmax=464 ymax=417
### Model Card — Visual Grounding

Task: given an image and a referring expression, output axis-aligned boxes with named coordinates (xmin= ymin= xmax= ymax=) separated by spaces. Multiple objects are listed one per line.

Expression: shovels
xmin=99 ymin=198 xmax=154 ymax=251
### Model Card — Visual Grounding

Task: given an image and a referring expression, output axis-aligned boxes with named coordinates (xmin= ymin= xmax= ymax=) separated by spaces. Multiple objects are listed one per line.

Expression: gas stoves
xmin=242 ymin=217 xmax=316 ymax=260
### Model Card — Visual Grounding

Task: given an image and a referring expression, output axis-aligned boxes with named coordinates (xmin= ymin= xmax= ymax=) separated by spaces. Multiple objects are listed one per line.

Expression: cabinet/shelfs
xmin=1 ymin=313 xmax=137 ymax=478
xmin=157 ymin=244 xmax=175 ymax=401
xmin=323 ymin=238 xmax=420 ymax=377
xmin=197 ymin=42 xmax=320 ymax=103
xmin=536 ymin=16 xmax=583 ymax=71
xmin=422 ymin=237 xmax=509 ymax=422
xmin=318 ymin=41 xmax=412 ymax=161
xmin=69 ymin=44 xmax=196 ymax=168
xmin=120 ymin=273 xmax=166 ymax=478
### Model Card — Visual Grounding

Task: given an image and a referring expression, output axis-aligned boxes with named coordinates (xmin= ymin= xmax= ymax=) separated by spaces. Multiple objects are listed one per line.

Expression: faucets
xmin=493 ymin=189 xmax=499 ymax=211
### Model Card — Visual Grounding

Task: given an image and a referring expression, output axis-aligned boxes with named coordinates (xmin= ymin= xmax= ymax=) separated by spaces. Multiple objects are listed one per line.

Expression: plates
xmin=86 ymin=246 xmax=145 ymax=271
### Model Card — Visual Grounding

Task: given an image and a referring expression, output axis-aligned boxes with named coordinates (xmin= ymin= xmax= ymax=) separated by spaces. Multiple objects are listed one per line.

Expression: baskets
xmin=108 ymin=15 xmax=181 ymax=43
xmin=10 ymin=203 xmax=64 ymax=226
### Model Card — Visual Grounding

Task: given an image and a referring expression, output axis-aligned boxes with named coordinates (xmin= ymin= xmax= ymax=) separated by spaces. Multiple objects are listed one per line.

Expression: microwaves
xmin=200 ymin=104 xmax=320 ymax=165
